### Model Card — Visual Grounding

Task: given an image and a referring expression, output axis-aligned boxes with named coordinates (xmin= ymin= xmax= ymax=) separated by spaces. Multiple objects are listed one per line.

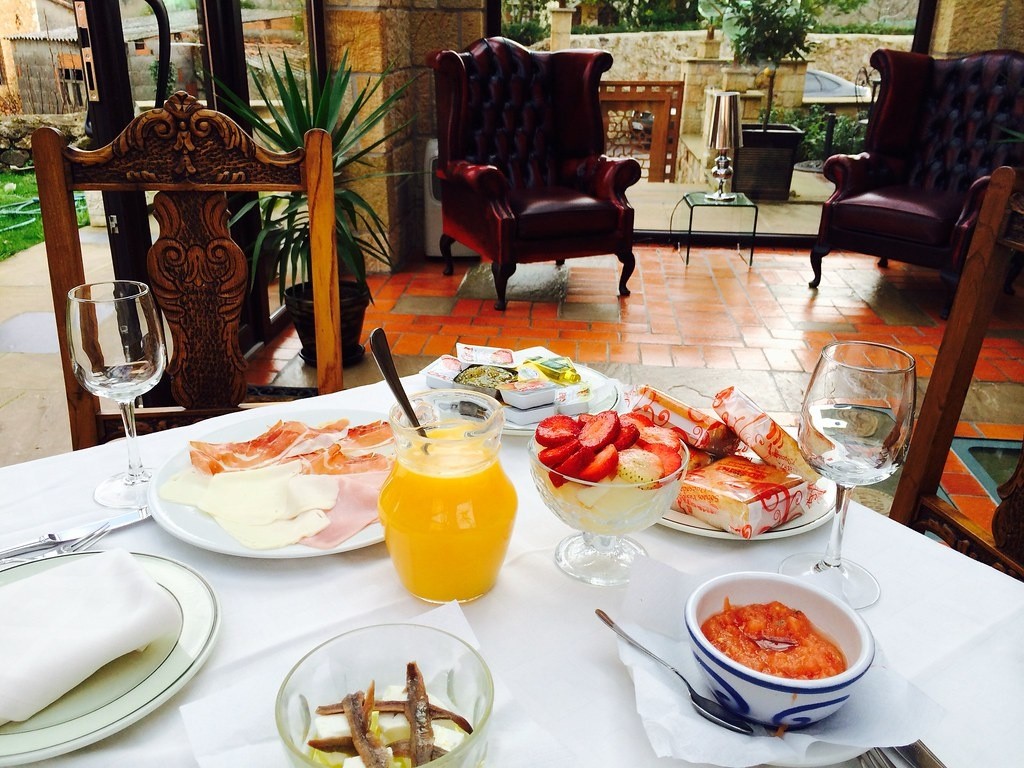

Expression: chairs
xmin=426 ymin=35 xmax=639 ymax=309
xmin=806 ymin=50 xmax=1024 ymax=288
xmin=884 ymin=165 xmax=1023 ymax=583
xmin=32 ymin=92 xmax=342 ymax=454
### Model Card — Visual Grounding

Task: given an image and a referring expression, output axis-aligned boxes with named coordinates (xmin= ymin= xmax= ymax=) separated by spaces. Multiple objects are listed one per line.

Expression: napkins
xmin=0 ymin=541 xmax=169 ymax=728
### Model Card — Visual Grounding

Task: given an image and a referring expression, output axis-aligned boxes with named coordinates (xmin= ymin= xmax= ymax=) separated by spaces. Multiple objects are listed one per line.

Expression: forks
xmin=0 ymin=521 xmax=111 ymax=564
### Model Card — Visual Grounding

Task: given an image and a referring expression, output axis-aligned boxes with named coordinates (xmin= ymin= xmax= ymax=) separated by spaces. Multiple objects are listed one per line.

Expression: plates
xmin=658 ymin=476 xmax=840 ymax=538
xmin=145 ymin=407 xmax=385 ymax=559
xmin=501 ymin=369 xmax=623 ymax=436
xmin=0 ymin=551 xmax=221 ymax=768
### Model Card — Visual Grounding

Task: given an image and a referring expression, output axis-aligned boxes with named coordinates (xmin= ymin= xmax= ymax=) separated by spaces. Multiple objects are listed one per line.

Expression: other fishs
xmin=311 ymin=736 xmax=448 ymax=761
xmin=316 ymin=696 xmax=474 ymax=735
xmin=404 ymin=661 xmax=435 ymax=768
xmin=343 ymin=679 xmax=392 ymax=768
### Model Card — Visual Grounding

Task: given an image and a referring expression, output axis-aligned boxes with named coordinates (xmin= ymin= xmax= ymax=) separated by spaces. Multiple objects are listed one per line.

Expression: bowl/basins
xmin=685 ymin=571 xmax=876 ymax=729
xmin=273 ymin=623 xmax=494 ymax=768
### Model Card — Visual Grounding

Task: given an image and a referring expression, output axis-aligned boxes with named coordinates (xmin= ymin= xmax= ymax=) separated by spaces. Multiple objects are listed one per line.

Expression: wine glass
xmin=526 ymin=419 xmax=690 ymax=584
xmin=779 ymin=341 xmax=916 ymax=607
xmin=67 ymin=281 xmax=174 ymax=511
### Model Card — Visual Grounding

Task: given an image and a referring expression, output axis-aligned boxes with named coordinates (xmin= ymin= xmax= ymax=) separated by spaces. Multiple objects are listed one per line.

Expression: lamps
xmin=703 ymin=90 xmax=746 ymax=201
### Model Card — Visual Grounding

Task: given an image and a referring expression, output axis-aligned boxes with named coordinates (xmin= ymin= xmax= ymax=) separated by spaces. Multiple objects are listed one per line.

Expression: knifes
xmin=0 ymin=507 xmax=151 ymax=561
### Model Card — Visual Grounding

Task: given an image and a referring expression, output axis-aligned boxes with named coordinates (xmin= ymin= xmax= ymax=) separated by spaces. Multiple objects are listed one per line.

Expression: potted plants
xmin=246 ymin=45 xmax=424 ymax=366
xmin=713 ymin=0 xmax=815 ymax=199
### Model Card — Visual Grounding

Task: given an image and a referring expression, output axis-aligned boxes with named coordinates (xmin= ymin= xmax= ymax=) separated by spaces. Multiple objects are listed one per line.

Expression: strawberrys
xmin=533 ymin=410 xmax=684 ymax=490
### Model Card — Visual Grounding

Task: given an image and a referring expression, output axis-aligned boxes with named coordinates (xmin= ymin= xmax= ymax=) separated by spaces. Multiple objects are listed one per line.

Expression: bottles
xmin=379 ymin=389 xmax=516 ymax=603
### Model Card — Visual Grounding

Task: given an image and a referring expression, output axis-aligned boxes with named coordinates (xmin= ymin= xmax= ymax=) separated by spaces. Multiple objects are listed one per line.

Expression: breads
xmin=631 ymin=384 xmax=822 ymax=539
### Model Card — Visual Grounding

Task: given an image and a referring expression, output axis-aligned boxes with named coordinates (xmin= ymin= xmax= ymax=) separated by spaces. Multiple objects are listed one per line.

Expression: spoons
xmin=595 ymin=606 xmax=754 ymax=736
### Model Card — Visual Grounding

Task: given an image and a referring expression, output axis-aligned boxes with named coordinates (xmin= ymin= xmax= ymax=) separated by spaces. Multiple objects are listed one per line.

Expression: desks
xmin=0 ymin=341 xmax=1024 ymax=768
xmin=672 ymin=189 xmax=759 ymax=265
